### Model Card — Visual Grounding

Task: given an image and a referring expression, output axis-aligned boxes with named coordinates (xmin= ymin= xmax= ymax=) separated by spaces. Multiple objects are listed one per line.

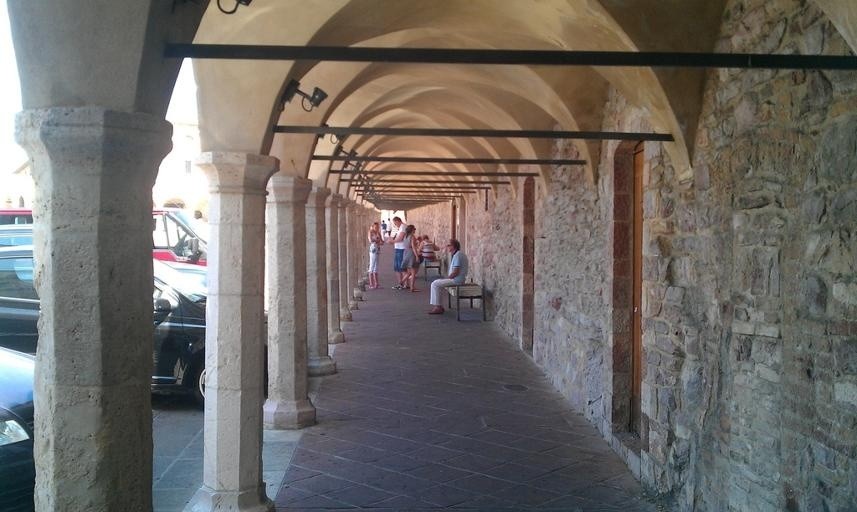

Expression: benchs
xmin=445 ymin=284 xmax=487 ymax=322
xmin=424 ymin=259 xmax=442 ymax=281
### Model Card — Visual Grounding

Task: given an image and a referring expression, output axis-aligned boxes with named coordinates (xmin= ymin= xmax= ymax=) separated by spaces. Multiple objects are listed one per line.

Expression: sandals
xmin=368 ymin=286 xmax=383 ymax=289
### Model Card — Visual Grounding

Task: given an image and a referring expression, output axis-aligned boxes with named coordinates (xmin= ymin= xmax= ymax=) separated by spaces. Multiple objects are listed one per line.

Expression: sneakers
xmin=391 ymin=284 xmax=410 ymax=290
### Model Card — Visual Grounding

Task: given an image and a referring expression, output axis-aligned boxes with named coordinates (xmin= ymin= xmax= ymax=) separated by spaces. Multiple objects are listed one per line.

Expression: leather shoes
xmin=428 ymin=307 xmax=445 ymax=314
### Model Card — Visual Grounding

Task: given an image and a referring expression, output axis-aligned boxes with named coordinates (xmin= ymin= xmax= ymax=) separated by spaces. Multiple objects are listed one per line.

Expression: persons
xmin=194 ymin=211 xmax=206 ymax=228
xmin=382 ymin=217 xmax=434 ymax=292
xmin=428 ymin=239 xmax=468 ymax=314
xmin=368 ymin=223 xmax=384 ymax=289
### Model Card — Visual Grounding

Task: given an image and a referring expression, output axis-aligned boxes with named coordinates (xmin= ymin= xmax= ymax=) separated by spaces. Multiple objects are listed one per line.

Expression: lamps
xmin=215 ymin=0 xmax=252 ymax=15
xmin=278 ymin=79 xmax=329 ymax=112
xmin=317 ymin=121 xmax=374 ymax=195
